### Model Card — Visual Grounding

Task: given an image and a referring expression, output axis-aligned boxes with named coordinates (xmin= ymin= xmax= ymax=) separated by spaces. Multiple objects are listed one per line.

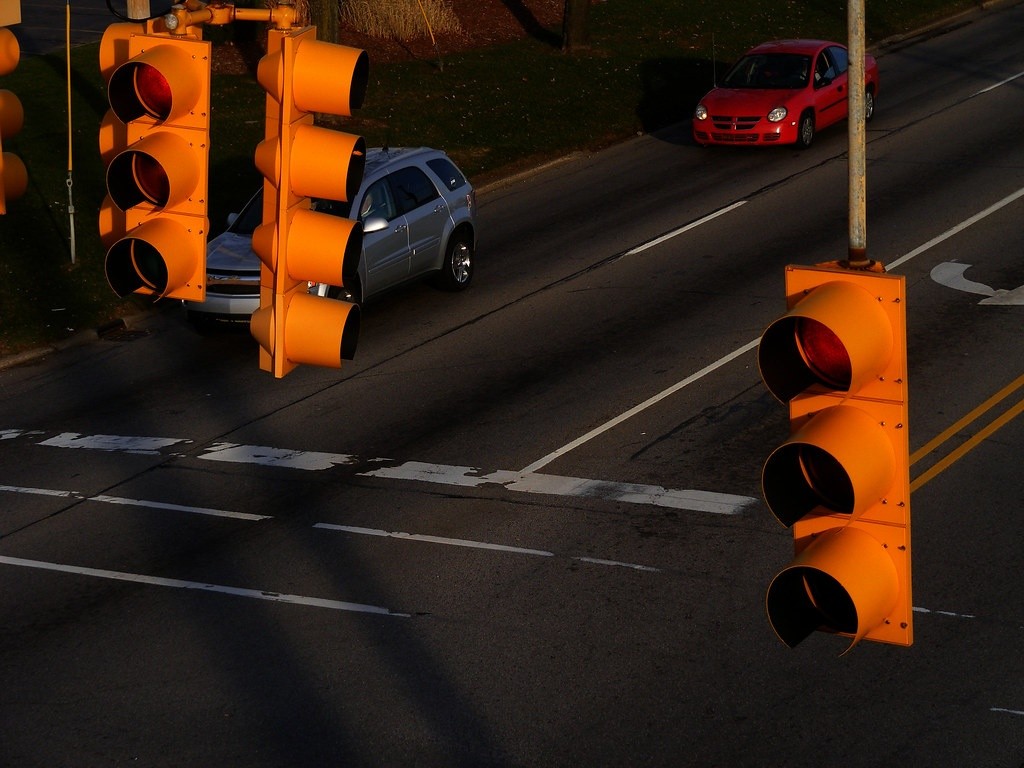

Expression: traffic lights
xmin=759 ymin=264 xmax=913 ymax=654
xmin=105 ymin=35 xmax=212 ymax=304
xmin=275 ymin=27 xmax=370 ymax=379
xmin=0 ymin=0 xmax=28 ymax=215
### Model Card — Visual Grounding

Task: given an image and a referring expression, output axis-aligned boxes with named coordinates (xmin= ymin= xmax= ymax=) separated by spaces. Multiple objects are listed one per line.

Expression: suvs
xmin=179 ymin=146 xmax=479 ymax=337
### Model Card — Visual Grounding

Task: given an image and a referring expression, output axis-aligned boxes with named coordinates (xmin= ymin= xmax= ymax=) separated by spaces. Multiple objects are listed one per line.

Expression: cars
xmin=692 ymin=39 xmax=879 ymax=150
